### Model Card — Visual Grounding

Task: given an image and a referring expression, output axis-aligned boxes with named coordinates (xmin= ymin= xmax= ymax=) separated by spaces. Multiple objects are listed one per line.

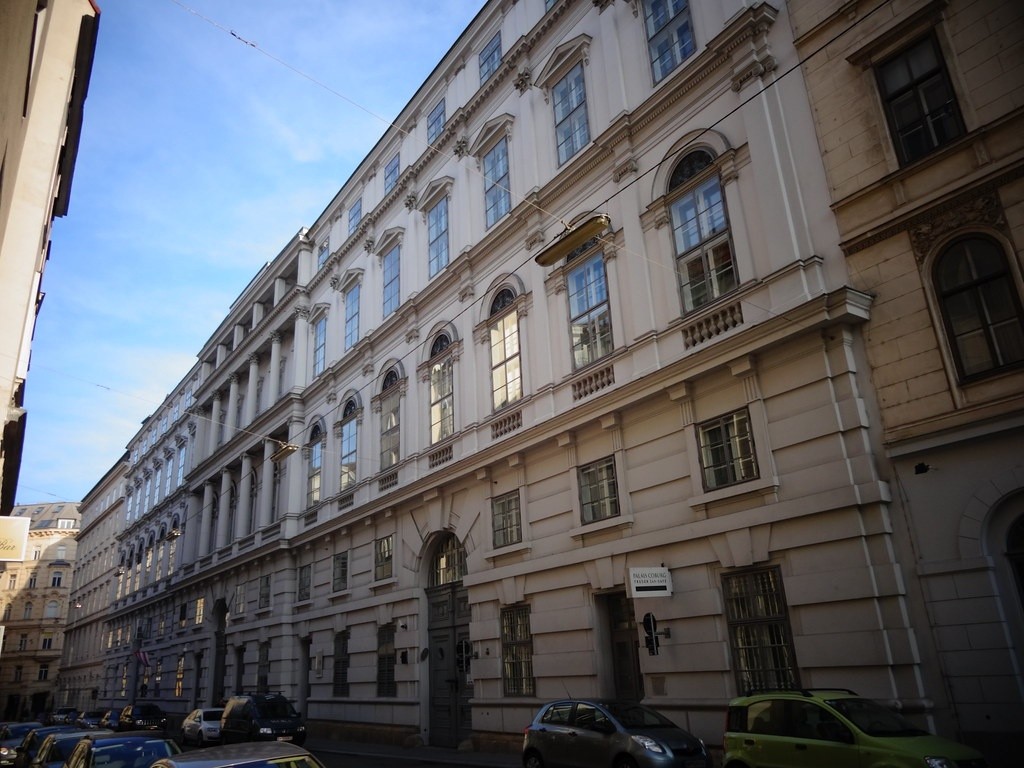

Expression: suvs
xmin=52 ymin=707 xmax=80 ymax=724
xmin=221 ymin=690 xmax=306 ymax=746
xmin=723 ymin=688 xmax=990 ymax=768
xmin=119 ymin=704 xmax=168 ymax=730
xmin=63 ymin=727 xmax=183 ymax=768
xmin=0 ymin=722 xmax=44 ymax=768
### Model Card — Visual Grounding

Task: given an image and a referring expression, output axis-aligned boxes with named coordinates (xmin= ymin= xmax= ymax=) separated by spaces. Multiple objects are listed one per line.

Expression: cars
xmin=180 ymin=708 xmax=225 ymax=748
xmin=101 ymin=710 xmax=120 ymax=728
xmin=76 ymin=711 xmax=103 ymax=728
xmin=33 ymin=733 xmax=82 ymax=768
xmin=14 ymin=726 xmax=86 ymax=768
xmin=522 ymin=700 xmax=714 ymax=768
xmin=151 ymin=742 xmax=325 ymax=768
